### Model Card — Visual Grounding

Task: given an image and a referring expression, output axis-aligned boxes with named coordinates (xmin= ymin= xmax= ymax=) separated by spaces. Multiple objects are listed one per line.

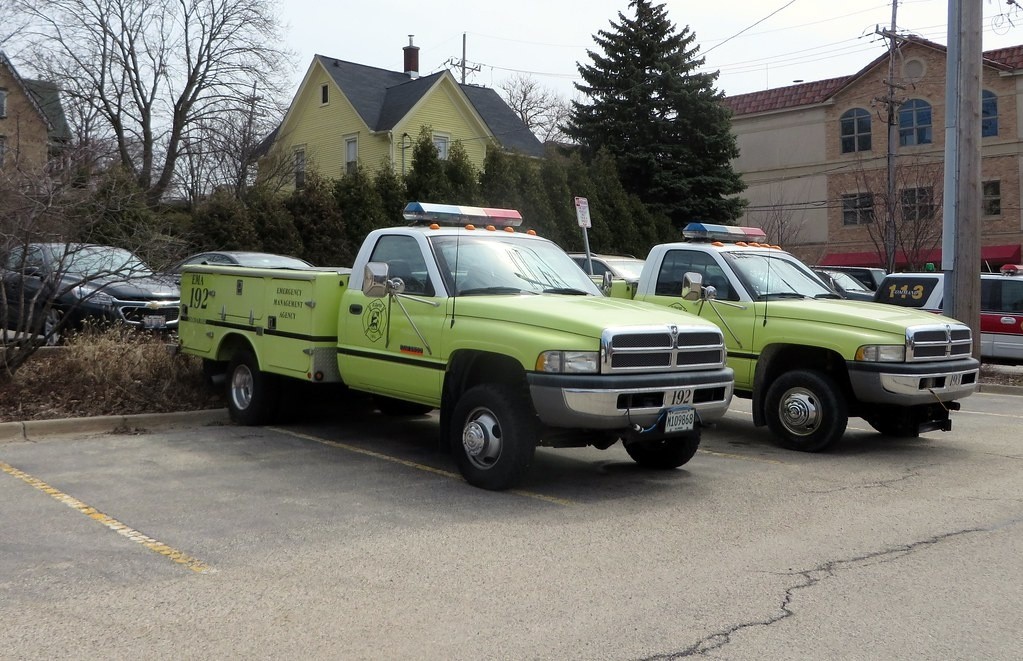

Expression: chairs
xmin=388 ymin=259 xmax=425 ymax=293
xmin=458 ymin=265 xmax=502 ymax=290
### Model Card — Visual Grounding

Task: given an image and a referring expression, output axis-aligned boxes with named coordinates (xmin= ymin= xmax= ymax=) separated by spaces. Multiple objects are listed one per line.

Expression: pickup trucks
xmin=411 ymin=222 xmax=980 ymax=454
xmin=164 ymin=200 xmax=737 ymax=492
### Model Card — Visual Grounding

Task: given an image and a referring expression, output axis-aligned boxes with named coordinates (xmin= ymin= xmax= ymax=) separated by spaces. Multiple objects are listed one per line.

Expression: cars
xmin=0 ymin=243 xmax=182 ymax=348
xmin=813 ymin=268 xmax=875 ymax=301
xmin=154 ymin=250 xmax=315 ymax=287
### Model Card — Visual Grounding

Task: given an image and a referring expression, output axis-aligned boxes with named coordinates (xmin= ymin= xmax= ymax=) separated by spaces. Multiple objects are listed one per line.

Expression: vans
xmin=872 ymin=264 xmax=1023 ymax=364
xmin=809 ymin=263 xmax=887 ymax=292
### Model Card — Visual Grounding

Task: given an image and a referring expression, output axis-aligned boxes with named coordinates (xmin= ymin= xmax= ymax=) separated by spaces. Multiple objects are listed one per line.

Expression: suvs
xmin=564 ymin=250 xmax=647 ymax=287
